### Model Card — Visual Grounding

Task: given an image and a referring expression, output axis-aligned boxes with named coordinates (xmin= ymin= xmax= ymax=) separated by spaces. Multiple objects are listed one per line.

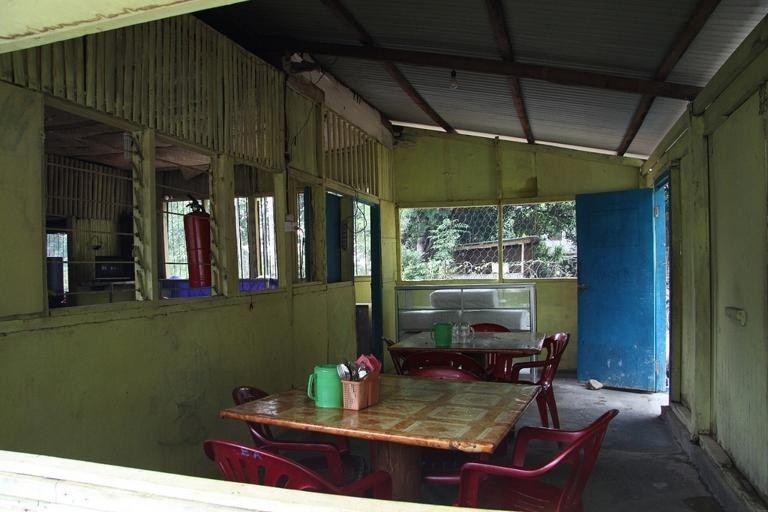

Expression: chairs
xmin=458 ymin=409 xmax=620 ymax=512
xmin=203 ymin=438 xmax=392 ymax=501
xmin=495 ymin=331 xmax=570 ymax=429
xmin=400 ymin=349 xmax=489 ymax=380
xmin=451 ymin=323 xmax=512 ymax=379
xmin=405 ymin=368 xmax=507 ymax=485
xmin=382 ymin=336 xmax=406 ymax=375
xmin=232 ymin=385 xmax=350 ymax=487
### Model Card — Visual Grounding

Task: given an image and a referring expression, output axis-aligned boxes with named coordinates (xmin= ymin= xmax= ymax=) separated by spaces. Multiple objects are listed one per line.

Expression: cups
xmin=460 ymin=321 xmax=474 ymax=343
xmin=307 ymin=363 xmax=343 ymax=409
xmin=430 ymin=321 xmax=452 ymax=348
xmin=448 ymin=319 xmax=459 ymax=342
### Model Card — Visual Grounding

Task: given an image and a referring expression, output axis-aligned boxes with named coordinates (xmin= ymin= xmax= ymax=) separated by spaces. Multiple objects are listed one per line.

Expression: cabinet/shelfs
xmin=394 ymin=285 xmax=536 ymax=382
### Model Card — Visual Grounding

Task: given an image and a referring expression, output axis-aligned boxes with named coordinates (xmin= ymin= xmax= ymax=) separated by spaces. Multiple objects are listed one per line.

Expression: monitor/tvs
xmin=94 ymin=256 xmax=128 ymax=278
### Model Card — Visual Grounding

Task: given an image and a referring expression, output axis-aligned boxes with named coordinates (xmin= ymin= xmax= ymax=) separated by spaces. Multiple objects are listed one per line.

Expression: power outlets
xmin=285 ymin=221 xmax=298 ymax=232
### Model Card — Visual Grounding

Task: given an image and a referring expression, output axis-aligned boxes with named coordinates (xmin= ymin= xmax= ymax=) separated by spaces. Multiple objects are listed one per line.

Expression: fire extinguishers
xmin=184 ymin=192 xmax=211 ymax=287
